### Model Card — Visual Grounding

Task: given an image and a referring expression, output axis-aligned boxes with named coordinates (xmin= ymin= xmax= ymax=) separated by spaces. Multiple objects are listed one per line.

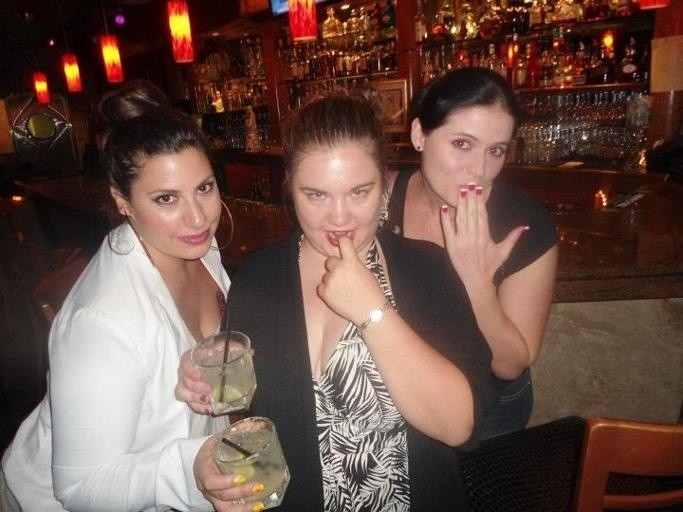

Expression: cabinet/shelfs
xmin=191 ymin=1 xmax=683 ymax=170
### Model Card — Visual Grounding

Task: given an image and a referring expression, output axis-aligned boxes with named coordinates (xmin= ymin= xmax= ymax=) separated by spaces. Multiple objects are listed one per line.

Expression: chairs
xmin=0 ymin=196 xmax=88 ymax=397
xmin=457 ymin=414 xmax=683 ymax=512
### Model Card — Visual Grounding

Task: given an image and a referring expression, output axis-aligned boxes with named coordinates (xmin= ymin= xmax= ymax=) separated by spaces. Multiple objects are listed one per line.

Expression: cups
xmin=190 ymin=331 xmax=259 ymax=417
xmin=210 ymin=416 xmax=292 ymax=510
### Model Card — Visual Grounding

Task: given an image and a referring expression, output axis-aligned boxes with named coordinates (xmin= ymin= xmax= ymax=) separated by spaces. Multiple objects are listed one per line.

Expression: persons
xmin=174 ymin=95 xmax=503 ymax=511
xmin=381 ymin=67 xmax=559 ymax=439
xmin=0 ymin=78 xmax=268 ymax=512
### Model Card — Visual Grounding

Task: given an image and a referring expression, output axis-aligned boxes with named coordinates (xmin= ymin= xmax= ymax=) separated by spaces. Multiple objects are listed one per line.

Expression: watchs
xmin=354 ymin=300 xmax=394 ymax=332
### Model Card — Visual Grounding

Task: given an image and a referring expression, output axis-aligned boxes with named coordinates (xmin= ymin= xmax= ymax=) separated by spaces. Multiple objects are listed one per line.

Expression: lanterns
xmin=32 ymin=70 xmax=50 ymax=105
xmin=61 ymin=52 xmax=82 ymax=93
xmin=166 ymin=0 xmax=195 ymax=64
xmin=289 ymin=0 xmax=319 ymax=42
xmin=98 ymin=33 xmax=126 ymax=83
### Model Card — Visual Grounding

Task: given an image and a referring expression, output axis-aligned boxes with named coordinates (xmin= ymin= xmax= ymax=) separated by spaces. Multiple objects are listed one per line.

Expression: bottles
xmin=287 ymin=0 xmax=649 ymax=164
